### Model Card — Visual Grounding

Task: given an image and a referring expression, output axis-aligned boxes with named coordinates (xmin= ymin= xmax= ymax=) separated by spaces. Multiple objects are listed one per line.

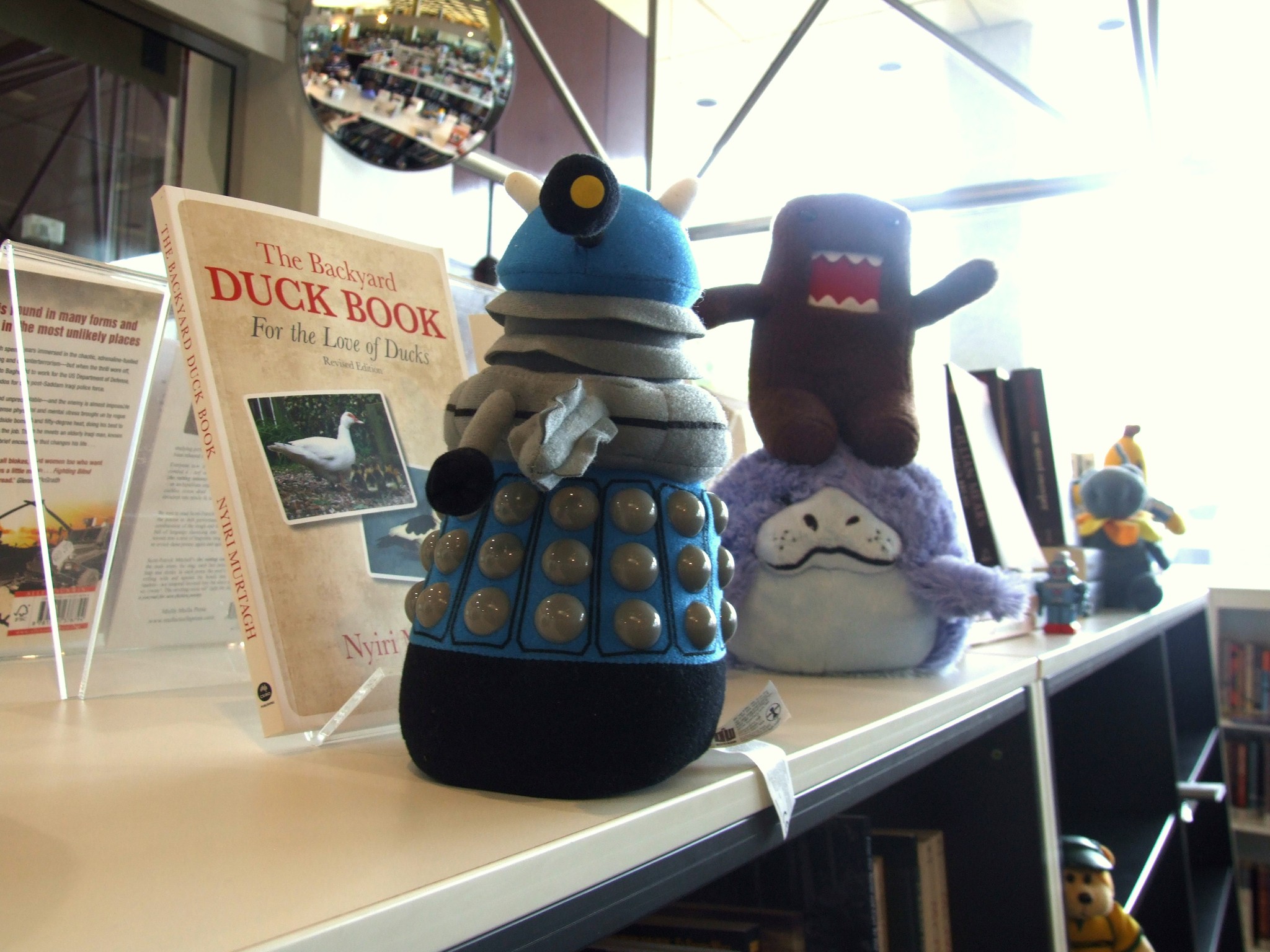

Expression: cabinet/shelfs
xmin=1046 ymin=607 xmax=1244 ymax=952
xmin=1208 ymin=588 xmax=1270 ymax=952
xmin=577 ymin=711 xmax=1053 ymax=951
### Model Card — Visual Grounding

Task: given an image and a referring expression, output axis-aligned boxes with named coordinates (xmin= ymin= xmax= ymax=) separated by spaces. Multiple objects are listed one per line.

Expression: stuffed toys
xmin=1076 ymin=424 xmax=1185 ymax=612
xmin=394 ymin=153 xmax=733 ymax=802
xmin=1059 ymin=834 xmax=1155 ymax=952
xmin=701 ymin=447 xmax=1035 ymax=676
xmin=680 ymin=194 xmax=998 ymax=470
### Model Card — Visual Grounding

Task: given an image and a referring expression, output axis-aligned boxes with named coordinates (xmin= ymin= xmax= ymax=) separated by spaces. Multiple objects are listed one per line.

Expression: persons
xmin=320 ymin=43 xmax=352 ymax=83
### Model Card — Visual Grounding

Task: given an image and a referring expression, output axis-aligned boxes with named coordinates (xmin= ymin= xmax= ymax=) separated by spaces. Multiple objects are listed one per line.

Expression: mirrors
xmin=298 ymin=0 xmax=516 ymax=173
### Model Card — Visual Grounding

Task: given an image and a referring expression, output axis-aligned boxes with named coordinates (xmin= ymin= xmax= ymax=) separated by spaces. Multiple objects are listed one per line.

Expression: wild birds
xmin=265 ymin=412 xmax=365 ymax=492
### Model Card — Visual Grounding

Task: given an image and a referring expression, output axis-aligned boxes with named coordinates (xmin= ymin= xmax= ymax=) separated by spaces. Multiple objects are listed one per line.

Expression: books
xmin=572 ymin=808 xmax=952 ymax=952
xmin=0 ymin=239 xmax=173 ymax=659
xmin=1217 ymin=640 xmax=1270 ymax=952
xmin=97 ymin=318 xmax=245 ymax=654
xmin=151 ymin=185 xmax=468 ymax=737
